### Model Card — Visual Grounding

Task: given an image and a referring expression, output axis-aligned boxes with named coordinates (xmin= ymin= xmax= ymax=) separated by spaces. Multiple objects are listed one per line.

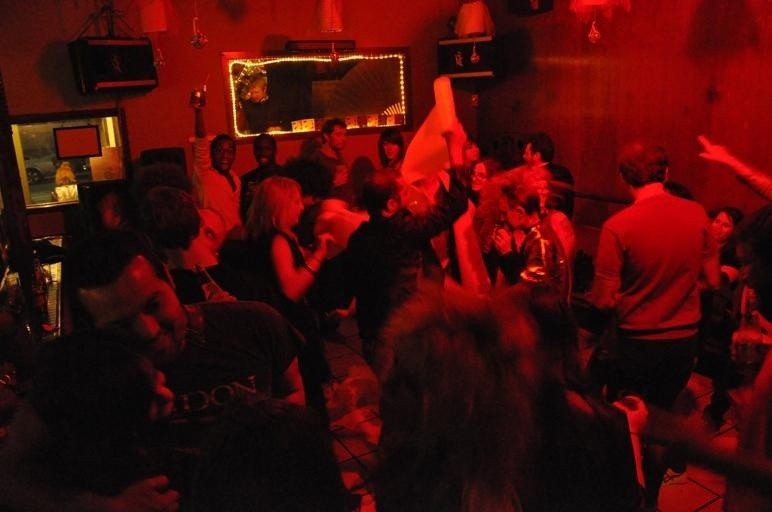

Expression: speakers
xmin=68 ymin=35 xmax=159 ymax=97
xmin=141 ymin=147 xmax=186 ymax=174
xmin=436 ymin=35 xmax=514 ymax=84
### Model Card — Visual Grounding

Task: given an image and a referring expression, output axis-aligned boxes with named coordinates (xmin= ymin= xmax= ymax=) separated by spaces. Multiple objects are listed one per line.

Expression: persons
xmin=242 ymin=77 xmax=285 ymax=135
xmin=0 ymin=119 xmax=771 ymax=512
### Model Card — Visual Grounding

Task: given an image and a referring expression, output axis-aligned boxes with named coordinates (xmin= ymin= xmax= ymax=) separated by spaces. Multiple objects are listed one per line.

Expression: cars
xmin=23 ymin=154 xmax=56 ymax=184
